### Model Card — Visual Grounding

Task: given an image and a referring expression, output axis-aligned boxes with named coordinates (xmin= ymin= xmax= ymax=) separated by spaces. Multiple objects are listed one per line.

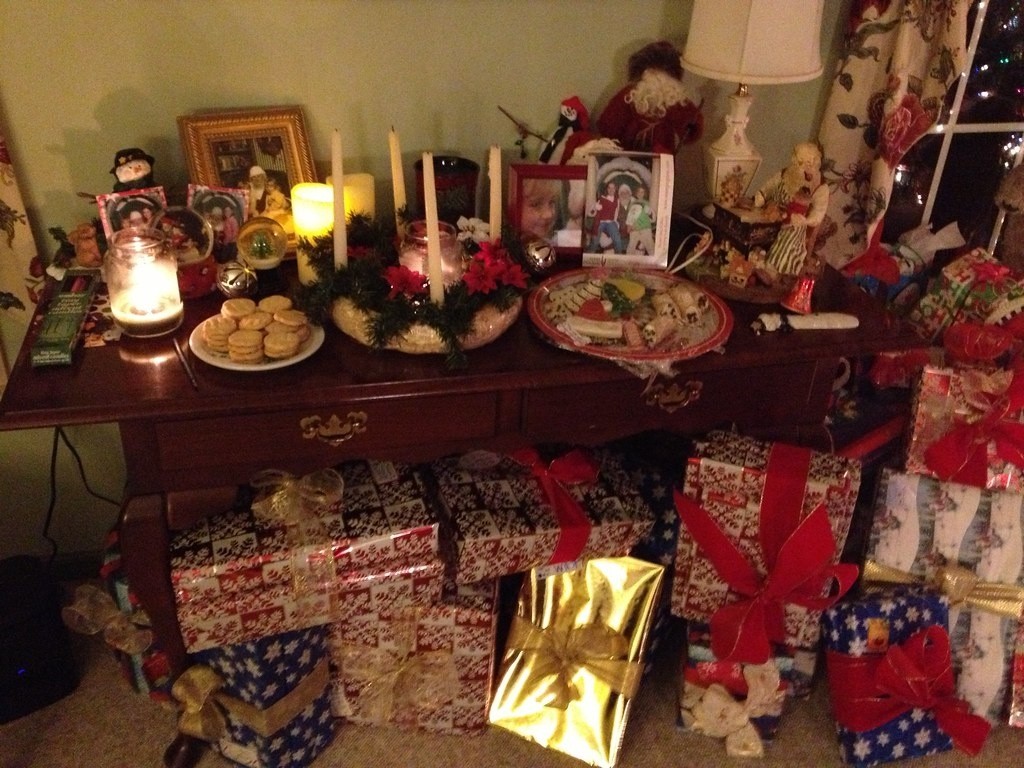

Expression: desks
xmin=0 ymin=218 xmax=925 ymax=677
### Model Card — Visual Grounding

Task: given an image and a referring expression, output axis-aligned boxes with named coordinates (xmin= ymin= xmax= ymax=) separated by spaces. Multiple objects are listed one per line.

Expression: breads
xmin=202 ymin=295 xmax=310 ymax=363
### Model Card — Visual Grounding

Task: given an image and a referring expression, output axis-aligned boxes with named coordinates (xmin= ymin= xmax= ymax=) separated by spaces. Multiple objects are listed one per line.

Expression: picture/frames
xmin=176 ymin=103 xmax=320 ymax=261
xmin=503 ymin=164 xmax=589 ymax=272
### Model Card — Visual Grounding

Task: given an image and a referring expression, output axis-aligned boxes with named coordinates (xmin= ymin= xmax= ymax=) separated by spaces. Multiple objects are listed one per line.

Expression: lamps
xmin=290 ymin=180 xmax=336 ymax=285
xmin=326 ymin=172 xmax=375 ymax=224
xmin=676 ymin=0 xmax=827 ymax=208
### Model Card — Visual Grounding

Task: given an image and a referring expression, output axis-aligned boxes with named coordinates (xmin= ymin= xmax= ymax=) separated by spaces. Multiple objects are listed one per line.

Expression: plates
xmin=528 ymin=269 xmax=733 ymax=363
xmin=190 ymin=313 xmax=324 ymax=371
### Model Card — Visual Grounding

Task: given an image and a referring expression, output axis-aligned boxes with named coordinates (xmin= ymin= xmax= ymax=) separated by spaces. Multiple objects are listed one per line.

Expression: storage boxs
xmin=62 ymin=245 xmax=1024 ymax=767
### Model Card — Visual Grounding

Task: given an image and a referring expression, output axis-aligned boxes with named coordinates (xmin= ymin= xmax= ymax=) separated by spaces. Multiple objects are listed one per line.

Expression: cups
xmin=414 ymin=155 xmax=480 ymax=221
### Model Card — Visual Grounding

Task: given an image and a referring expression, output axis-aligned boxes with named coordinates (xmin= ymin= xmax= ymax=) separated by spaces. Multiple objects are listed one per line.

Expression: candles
xmin=422 ymin=151 xmax=443 ymax=305
xmin=388 ymin=123 xmax=410 ymax=238
xmin=489 ymin=141 xmax=502 ymax=245
xmin=329 ymin=126 xmax=349 ymax=273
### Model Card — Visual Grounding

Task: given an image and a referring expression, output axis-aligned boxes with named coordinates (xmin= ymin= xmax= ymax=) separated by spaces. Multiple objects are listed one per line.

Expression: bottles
xmin=104 ymin=226 xmax=183 ymax=339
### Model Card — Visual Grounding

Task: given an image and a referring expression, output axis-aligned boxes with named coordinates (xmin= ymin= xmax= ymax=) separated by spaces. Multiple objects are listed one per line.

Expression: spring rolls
xmin=623 ymin=282 xmax=709 ymax=351
